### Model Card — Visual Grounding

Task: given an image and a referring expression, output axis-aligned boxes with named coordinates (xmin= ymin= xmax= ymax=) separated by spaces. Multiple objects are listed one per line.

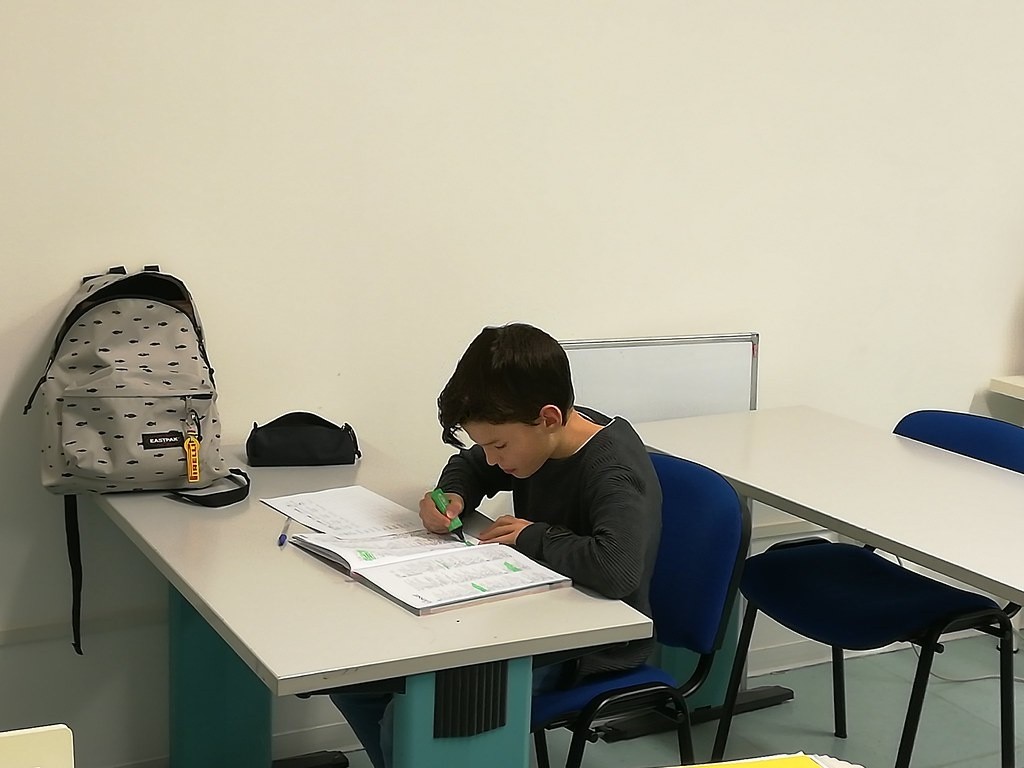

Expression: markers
xmin=429 ymin=488 xmax=468 ymax=543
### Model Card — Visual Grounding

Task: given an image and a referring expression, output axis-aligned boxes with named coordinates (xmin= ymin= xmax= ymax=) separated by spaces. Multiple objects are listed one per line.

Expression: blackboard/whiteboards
xmin=559 ymin=334 xmax=763 ymax=419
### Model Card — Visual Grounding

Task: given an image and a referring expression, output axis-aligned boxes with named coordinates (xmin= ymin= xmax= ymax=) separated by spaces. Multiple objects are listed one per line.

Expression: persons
xmin=328 ymin=323 xmax=661 ymax=768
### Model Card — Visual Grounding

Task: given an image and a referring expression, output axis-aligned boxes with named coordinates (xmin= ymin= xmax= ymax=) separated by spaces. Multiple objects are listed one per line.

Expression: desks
xmin=91 ymin=443 xmax=654 ymax=768
xmin=631 ymin=403 xmax=1024 ymax=768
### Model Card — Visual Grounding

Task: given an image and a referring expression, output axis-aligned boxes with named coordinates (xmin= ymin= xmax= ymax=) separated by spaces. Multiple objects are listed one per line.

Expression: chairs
xmin=710 ymin=409 xmax=1024 ymax=768
xmin=530 ymin=451 xmax=753 ymax=768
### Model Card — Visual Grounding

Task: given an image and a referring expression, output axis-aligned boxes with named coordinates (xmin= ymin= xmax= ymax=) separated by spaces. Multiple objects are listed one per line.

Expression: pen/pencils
xmin=278 ymin=516 xmax=292 ymax=546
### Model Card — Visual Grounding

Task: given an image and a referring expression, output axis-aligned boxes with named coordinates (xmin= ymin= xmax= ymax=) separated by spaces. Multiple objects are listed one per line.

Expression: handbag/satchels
xmin=244 ymin=409 xmax=363 ymax=468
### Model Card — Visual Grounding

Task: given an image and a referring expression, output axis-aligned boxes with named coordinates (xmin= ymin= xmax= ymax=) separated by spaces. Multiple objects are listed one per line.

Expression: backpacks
xmin=22 ymin=263 xmax=251 ymax=504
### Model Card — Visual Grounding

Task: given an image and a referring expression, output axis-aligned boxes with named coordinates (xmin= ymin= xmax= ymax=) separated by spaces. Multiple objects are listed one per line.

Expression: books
xmin=289 ymin=532 xmax=573 ymax=616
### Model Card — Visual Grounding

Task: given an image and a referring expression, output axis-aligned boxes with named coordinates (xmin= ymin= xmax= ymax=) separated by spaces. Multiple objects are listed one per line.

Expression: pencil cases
xmin=245 ymin=410 xmax=364 ymax=467
